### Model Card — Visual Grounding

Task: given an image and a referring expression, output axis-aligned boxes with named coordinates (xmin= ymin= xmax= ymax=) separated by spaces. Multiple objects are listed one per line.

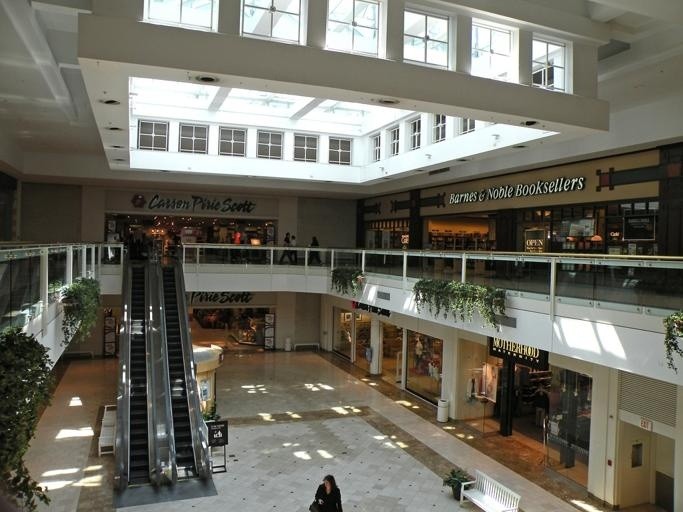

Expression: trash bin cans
xmin=560 ymin=444 xmax=575 ymax=468
xmin=437 ymin=399 xmax=449 ymax=423
xmin=284 ymin=336 xmax=291 ymax=352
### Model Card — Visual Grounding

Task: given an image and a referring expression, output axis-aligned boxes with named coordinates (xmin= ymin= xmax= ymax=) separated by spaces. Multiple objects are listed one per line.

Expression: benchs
xmin=97 ymin=403 xmax=119 ymax=455
xmin=458 ymin=469 xmax=520 ymax=512
xmin=59 ymin=348 xmax=95 ymax=360
xmin=291 ymin=342 xmax=320 ymax=352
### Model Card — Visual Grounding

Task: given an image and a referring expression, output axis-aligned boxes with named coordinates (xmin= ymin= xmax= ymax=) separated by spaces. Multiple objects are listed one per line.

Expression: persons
xmin=314 ymin=475 xmax=343 ymax=512
xmin=307 ymin=235 xmax=322 ymax=266
xmin=290 ymin=234 xmax=298 ymax=263
xmin=278 ymin=232 xmax=295 ymax=265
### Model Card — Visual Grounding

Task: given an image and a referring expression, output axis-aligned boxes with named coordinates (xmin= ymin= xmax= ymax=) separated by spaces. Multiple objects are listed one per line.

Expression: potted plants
xmin=60 ymin=276 xmax=104 ymax=346
xmin=443 ymin=467 xmax=474 ymax=501
xmin=330 ymin=269 xmax=366 ymax=297
xmin=412 ymin=278 xmax=506 ymax=332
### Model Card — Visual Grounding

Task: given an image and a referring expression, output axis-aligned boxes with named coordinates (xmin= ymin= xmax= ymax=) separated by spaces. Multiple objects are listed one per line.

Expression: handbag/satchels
xmin=309 ymin=500 xmax=323 ymax=512
